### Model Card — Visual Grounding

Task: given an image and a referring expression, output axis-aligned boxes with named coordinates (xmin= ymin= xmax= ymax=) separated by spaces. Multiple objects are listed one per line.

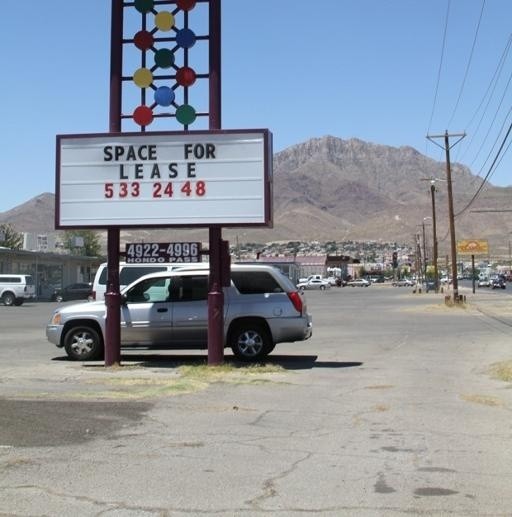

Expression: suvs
xmin=0 ymin=272 xmax=38 ymax=306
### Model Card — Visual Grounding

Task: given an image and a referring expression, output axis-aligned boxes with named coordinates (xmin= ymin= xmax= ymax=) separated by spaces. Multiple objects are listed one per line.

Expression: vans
xmin=44 ymin=259 xmax=315 ymax=365
xmin=89 ymin=257 xmax=216 ymax=304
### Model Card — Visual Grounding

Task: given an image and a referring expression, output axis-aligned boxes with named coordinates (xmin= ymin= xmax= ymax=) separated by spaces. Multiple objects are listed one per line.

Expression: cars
xmin=293 ymin=267 xmax=512 ymax=291
xmin=50 ymin=280 xmax=93 ymax=303
